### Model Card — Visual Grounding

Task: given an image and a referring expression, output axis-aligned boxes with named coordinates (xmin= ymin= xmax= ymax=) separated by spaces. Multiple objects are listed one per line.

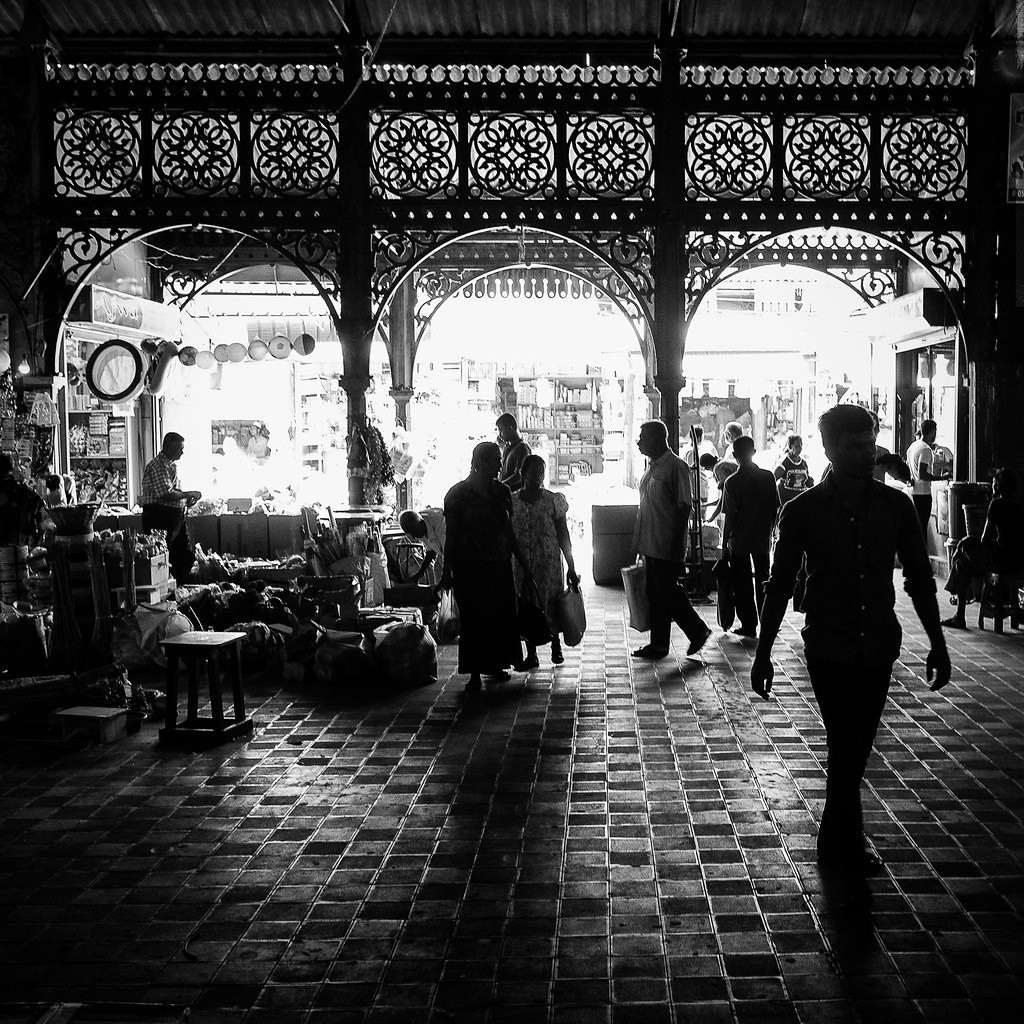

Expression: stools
xmin=156 ymin=631 xmax=252 ymax=749
xmin=978 ymin=573 xmax=1020 ymax=632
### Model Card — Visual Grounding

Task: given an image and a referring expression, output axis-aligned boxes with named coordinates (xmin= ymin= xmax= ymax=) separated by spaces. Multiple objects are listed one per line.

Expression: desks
xmin=111 ymin=579 xmax=177 ymax=608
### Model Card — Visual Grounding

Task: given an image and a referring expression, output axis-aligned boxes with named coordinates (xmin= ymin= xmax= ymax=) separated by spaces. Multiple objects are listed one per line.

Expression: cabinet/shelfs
xmin=71 ymin=409 xmax=138 ymax=509
xmin=518 ymin=377 xmax=604 ymax=484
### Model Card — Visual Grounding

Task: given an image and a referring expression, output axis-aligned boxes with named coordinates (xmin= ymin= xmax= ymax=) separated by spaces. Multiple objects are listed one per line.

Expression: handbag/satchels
xmin=516 ymin=577 xmax=554 ymax=644
xmin=710 ymin=558 xmax=735 ymax=629
xmin=436 ymin=587 xmax=461 ymax=642
xmin=621 ymin=553 xmax=674 ymax=632
xmin=559 ymin=581 xmax=587 ymax=646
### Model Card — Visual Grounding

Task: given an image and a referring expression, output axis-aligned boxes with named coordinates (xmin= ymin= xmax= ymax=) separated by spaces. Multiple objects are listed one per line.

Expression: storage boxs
xmin=267 ymin=510 xmax=320 ymax=560
xmin=110 ymin=444 xmax=124 ymax=454
xmin=94 ymin=518 xmax=117 ymax=539
xmin=108 ymin=426 xmax=125 ymax=444
xmin=183 ymin=512 xmax=220 ymax=555
xmin=135 ymin=551 xmax=169 ymax=584
xmin=86 ymin=435 xmax=108 ymax=454
xmin=53 ymin=706 xmax=129 ymax=746
xmin=118 ymin=514 xmax=143 ymax=534
xmin=219 ymin=511 xmax=268 ymax=560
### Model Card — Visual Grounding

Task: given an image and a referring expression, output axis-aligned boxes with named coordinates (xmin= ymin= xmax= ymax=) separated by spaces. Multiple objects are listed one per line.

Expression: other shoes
xmin=550 ymin=645 xmax=564 ymax=664
xmin=521 ymin=657 xmax=539 ymax=670
xmin=686 ymin=629 xmax=712 ymax=656
xmin=940 ymin=616 xmax=966 ymax=629
xmin=493 ymin=670 xmax=511 ymax=682
xmin=465 ymin=678 xmax=483 ymax=692
xmin=632 ymin=646 xmax=669 ymax=657
xmin=734 ymin=629 xmax=757 ymax=640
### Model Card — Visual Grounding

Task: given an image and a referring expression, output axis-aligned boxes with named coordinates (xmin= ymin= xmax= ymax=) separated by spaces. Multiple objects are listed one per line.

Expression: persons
xmin=906 ymin=419 xmax=950 ymax=523
xmin=438 ymin=442 xmax=523 ymax=688
xmin=495 ymin=413 xmax=531 ymax=491
xmin=724 ymin=422 xmax=742 ymax=463
xmin=685 ymin=424 xmax=719 ymax=519
xmin=398 ymin=507 xmax=446 ymax=582
xmin=42 ymin=474 xmax=76 ymax=505
xmin=914 ymin=430 xmax=953 ymax=476
xmin=700 ymin=453 xmax=738 ymax=523
xmin=140 ymin=432 xmax=203 ymax=586
xmin=940 ymin=467 xmax=1024 ymax=628
xmin=751 ymin=403 xmax=952 ymax=873
xmin=507 ymin=455 xmax=580 ymax=670
xmin=820 ymin=411 xmax=911 ymax=484
xmin=247 ymin=421 xmax=267 ymax=458
xmin=630 ymin=420 xmax=711 ymax=658
xmin=772 ymin=434 xmax=815 ymax=502
xmin=720 ymin=436 xmax=782 ymax=638
xmin=0 ymin=451 xmax=44 ymax=546
